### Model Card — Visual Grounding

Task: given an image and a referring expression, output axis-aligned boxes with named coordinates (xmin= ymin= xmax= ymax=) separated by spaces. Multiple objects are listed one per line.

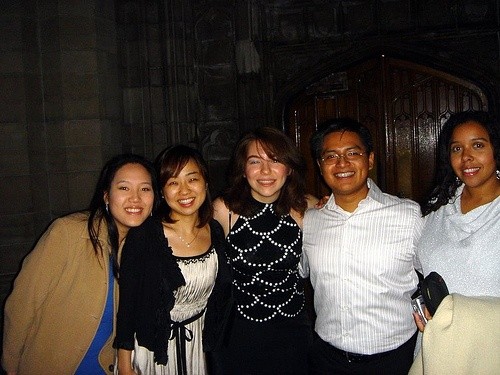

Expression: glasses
xmin=321 ymin=150 xmax=366 ymax=164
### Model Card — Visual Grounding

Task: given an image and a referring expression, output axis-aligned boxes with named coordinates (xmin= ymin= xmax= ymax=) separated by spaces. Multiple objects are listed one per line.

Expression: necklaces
xmin=170 ymin=224 xmax=200 ymax=247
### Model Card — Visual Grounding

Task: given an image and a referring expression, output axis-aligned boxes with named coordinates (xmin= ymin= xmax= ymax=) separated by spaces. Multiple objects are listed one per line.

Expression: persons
xmin=116 ymin=143 xmax=228 ymax=375
xmin=202 ymin=127 xmax=329 ymax=375
xmin=299 ymin=116 xmax=426 ymax=375
xmin=0 ymin=153 xmax=159 ymax=375
xmin=413 ymin=109 xmax=500 ymax=358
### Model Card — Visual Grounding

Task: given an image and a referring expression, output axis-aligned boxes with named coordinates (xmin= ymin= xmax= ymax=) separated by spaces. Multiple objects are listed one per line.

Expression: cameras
xmin=411 ymin=294 xmax=428 ymax=325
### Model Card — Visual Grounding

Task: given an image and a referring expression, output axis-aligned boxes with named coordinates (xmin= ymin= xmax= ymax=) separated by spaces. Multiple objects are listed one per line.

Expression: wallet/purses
xmin=420 ymin=272 xmax=449 ymax=317
